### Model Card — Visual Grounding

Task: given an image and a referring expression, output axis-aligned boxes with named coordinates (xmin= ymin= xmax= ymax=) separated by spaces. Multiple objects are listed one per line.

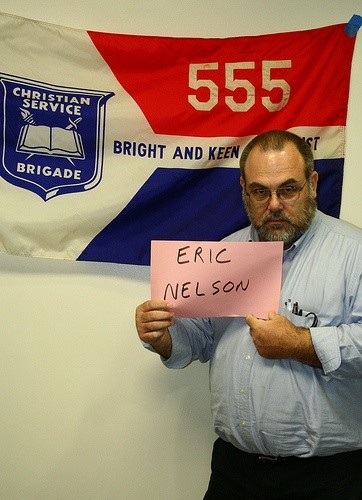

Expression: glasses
xmin=245 ymin=179 xmax=309 ymax=205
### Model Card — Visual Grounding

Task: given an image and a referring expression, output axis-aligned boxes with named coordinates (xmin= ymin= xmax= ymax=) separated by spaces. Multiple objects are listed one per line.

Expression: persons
xmin=133 ymin=128 xmax=361 ymax=500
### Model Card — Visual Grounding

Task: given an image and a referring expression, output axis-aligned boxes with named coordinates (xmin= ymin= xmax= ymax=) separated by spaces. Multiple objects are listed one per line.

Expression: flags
xmin=0 ymin=10 xmax=360 ymax=269
